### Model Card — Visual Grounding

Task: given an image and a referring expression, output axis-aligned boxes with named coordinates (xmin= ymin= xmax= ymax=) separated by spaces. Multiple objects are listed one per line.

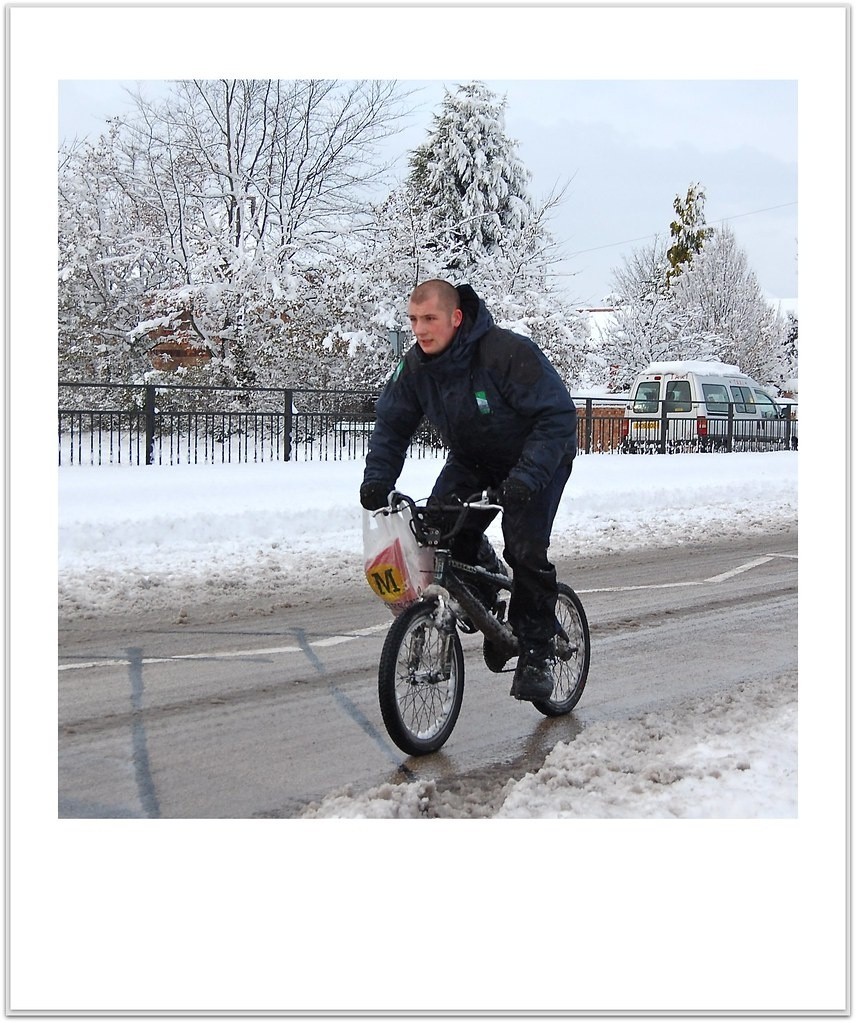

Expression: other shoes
xmin=457 ymin=558 xmax=507 ymax=633
xmin=511 ymin=642 xmax=556 ymax=701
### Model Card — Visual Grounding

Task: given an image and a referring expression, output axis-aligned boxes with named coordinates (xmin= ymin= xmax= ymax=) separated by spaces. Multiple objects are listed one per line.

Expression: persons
xmin=359 ymin=279 xmax=578 ymax=701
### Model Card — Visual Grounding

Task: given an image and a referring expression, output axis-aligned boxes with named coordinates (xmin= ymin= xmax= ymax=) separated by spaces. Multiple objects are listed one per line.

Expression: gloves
xmin=360 ymin=484 xmax=396 ymax=510
xmin=496 ymin=478 xmax=531 ymax=514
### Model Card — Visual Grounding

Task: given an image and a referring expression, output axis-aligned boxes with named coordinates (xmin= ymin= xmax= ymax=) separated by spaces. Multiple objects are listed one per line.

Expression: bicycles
xmin=371 ymin=488 xmax=591 ymax=756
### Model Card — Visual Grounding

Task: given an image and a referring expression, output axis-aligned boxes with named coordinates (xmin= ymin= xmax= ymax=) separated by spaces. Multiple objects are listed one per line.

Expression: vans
xmin=622 ymin=372 xmax=798 ymax=454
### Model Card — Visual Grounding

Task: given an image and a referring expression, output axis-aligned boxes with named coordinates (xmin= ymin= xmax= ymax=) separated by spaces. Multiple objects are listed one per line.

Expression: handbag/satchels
xmin=361 ymin=491 xmax=435 ymax=618
